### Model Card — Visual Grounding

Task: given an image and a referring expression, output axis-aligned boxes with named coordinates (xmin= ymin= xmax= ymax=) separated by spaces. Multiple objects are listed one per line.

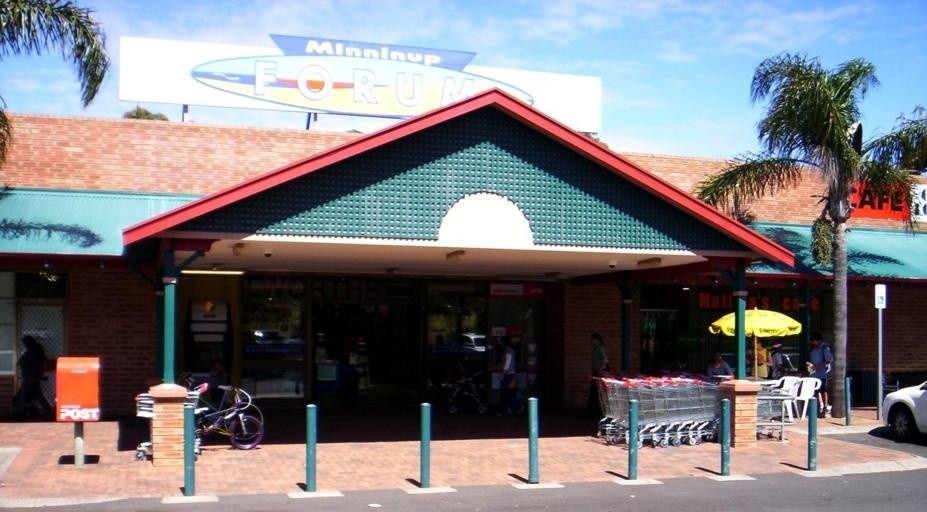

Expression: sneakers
xmin=817 ymin=408 xmax=826 ymax=417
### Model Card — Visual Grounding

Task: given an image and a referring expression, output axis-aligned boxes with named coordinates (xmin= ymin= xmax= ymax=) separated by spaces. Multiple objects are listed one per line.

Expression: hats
xmin=772 ymin=342 xmax=780 ymax=347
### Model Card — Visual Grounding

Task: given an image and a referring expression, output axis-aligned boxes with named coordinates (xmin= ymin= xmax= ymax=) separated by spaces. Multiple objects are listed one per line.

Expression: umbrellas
xmin=706 ymin=307 xmax=802 ymax=379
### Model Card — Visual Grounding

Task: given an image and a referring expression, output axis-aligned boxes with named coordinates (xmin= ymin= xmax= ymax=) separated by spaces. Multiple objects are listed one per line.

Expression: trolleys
xmin=441 ymin=362 xmax=498 ymax=417
xmin=591 ymin=371 xmax=791 ymax=450
xmin=133 ymin=381 xmax=209 ymax=462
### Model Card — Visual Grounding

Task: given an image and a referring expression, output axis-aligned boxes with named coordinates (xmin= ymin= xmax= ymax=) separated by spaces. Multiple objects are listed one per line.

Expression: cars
xmin=251 ymin=328 xmax=285 ymax=344
xmin=881 ymin=379 xmax=927 ymax=442
xmin=448 ymin=332 xmax=493 ymax=369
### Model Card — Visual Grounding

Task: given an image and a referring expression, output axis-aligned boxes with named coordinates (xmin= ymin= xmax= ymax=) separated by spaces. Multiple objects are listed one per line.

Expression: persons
xmin=586 ymin=332 xmax=610 ymax=417
xmin=804 ymin=333 xmax=833 ymax=414
xmin=13 ymin=335 xmax=55 ymax=422
xmin=768 ymin=336 xmax=795 ymax=380
xmin=209 ymin=354 xmax=229 ymax=406
xmin=746 ymin=336 xmax=774 ymax=379
xmin=494 ymin=334 xmax=524 ymax=417
xmin=704 ymin=348 xmax=735 ymax=384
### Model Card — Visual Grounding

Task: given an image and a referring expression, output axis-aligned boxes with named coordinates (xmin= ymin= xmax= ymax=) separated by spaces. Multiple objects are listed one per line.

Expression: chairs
xmin=770 ymin=376 xmax=800 ymax=421
xmin=781 ymin=377 xmax=821 ymax=423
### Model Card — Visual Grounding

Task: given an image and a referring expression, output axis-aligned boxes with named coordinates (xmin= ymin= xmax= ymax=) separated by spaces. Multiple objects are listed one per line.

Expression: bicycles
xmin=184 ymin=371 xmax=265 ymax=451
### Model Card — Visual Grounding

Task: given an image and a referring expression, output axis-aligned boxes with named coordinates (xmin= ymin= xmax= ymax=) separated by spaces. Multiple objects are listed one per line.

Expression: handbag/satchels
xmin=768 ymin=352 xmax=778 ymax=377
